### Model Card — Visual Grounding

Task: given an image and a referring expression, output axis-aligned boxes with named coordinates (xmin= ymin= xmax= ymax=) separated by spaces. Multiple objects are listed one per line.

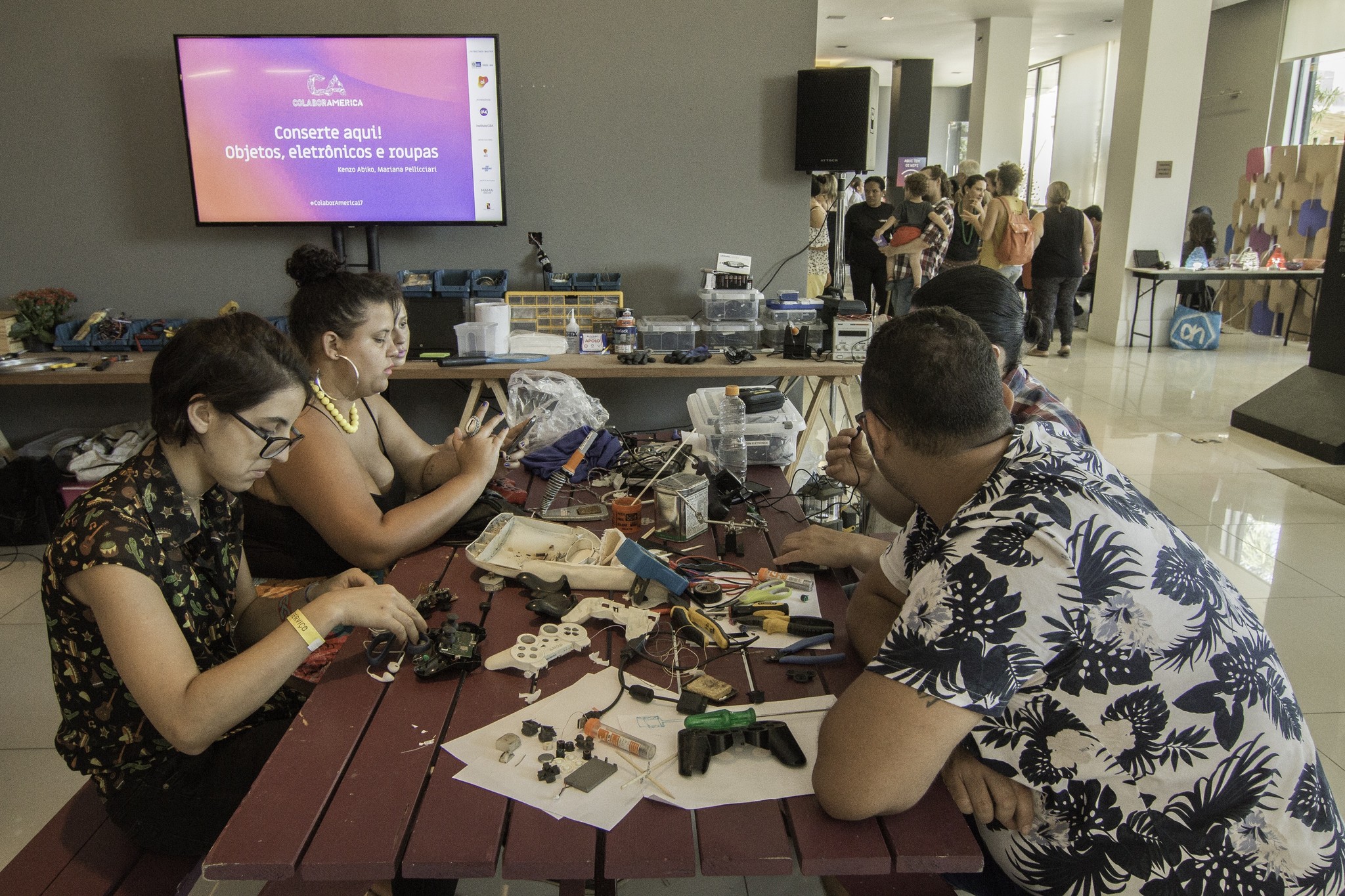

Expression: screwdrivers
xmin=684 ymin=707 xmax=831 ymax=729
xmin=703 ymin=601 xmax=789 ymax=619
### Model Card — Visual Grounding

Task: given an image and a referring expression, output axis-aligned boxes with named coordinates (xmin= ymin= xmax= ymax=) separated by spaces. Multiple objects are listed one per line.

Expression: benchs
xmin=0 ymin=778 xmax=211 ymax=896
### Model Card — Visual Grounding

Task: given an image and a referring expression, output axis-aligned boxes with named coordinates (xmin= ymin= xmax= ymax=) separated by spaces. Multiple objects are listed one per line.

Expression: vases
xmin=25 ymin=339 xmax=53 ymax=353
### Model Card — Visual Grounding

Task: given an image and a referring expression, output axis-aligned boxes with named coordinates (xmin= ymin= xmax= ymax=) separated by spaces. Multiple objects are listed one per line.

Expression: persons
xmin=778 ymin=159 xmax=1218 ymax=582
xmin=42 ymin=312 xmax=628 ymax=896
xmin=238 ymin=241 xmax=511 ymax=700
xmin=811 ymin=308 xmax=1345 ymax=896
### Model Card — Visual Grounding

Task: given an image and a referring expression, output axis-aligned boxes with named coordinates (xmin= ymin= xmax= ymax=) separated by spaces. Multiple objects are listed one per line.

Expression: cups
xmin=611 ymin=497 xmax=642 ymax=533
xmin=475 ymin=302 xmax=510 ymax=354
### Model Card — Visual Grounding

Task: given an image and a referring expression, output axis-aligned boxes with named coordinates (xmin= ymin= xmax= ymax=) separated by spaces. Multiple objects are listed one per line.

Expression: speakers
xmin=796 ymin=65 xmax=881 ymax=173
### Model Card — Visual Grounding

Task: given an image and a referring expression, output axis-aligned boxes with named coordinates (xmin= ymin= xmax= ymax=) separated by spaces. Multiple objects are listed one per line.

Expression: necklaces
xmin=311 ymin=379 xmax=359 ymax=434
xmin=960 ymin=200 xmax=977 ymax=246
xmin=184 ymin=491 xmax=205 ymax=501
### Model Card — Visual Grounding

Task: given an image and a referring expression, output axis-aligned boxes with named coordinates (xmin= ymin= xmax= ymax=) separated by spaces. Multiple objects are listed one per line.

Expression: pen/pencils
xmin=406 ymin=360 xmax=437 ymax=362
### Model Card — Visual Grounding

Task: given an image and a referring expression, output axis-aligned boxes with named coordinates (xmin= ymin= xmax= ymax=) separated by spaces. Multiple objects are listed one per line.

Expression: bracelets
xmin=305 ymin=584 xmax=318 ymax=603
xmin=279 ymin=590 xmax=294 ymax=624
xmin=285 ymin=608 xmax=326 ymax=651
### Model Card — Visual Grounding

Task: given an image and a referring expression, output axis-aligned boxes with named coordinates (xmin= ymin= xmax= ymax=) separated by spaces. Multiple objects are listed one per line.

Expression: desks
xmin=1126 ymin=268 xmax=1322 ymax=353
xmin=198 ymin=468 xmax=999 ymax=896
xmin=0 ymin=345 xmax=868 ymax=473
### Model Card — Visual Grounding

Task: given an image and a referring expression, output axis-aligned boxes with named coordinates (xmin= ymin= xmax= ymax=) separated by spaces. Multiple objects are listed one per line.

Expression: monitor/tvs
xmin=173 ymin=33 xmax=508 ymax=227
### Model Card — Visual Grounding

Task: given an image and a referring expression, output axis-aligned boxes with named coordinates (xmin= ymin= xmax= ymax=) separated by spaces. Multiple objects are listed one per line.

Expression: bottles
xmin=719 ymin=385 xmax=747 ymax=483
xmin=565 ymin=308 xmax=580 ymax=354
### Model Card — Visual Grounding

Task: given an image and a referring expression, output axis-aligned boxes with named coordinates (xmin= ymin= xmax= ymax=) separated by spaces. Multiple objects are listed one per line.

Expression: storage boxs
xmin=686 ymin=386 xmax=806 ymax=465
xmin=56 ymin=320 xmax=182 ymax=351
xmin=393 ymin=268 xmax=866 ymax=358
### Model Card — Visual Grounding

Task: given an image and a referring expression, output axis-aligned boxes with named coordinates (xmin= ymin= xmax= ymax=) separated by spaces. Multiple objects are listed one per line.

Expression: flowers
xmin=5 ymin=286 xmax=77 ymax=343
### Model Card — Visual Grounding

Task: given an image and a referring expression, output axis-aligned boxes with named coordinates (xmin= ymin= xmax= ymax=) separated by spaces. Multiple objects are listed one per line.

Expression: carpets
xmin=1263 ymin=467 xmax=1344 ymax=505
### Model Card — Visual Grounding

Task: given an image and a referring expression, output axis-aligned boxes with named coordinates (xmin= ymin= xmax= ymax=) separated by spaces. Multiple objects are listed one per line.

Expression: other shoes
xmin=1026 ymin=349 xmax=1050 ymax=358
xmin=1073 ymin=305 xmax=1084 ymax=317
xmin=1057 ymin=346 xmax=1071 ymax=356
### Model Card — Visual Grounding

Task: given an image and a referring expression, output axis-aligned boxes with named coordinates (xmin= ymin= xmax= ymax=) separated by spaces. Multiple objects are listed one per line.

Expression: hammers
xmin=96 ymin=354 xmax=129 ymax=373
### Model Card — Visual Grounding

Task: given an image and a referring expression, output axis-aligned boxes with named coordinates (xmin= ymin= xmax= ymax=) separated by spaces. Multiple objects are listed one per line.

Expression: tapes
xmin=693 ymin=582 xmax=722 ymax=604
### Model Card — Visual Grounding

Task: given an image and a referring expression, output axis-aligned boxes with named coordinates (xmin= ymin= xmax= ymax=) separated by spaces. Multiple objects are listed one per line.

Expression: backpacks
xmin=993 ymin=195 xmax=1034 ymax=266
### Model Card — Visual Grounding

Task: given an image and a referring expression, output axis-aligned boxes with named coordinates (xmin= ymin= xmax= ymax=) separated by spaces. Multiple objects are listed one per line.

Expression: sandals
xmin=885 ymin=276 xmax=895 ymax=291
xmin=907 ymin=285 xmax=921 ymax=301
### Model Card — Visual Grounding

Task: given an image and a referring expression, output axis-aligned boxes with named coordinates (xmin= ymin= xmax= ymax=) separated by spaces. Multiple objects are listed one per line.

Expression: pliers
xmin=50 ymin=362 xmax=88 ymax=369
xmin=762 ymin=633 xmax=846 ymax=664
xmin=735 ymin=615 xmax=835 ymax=637
xmin=668 ymin=592 xmax=730 ymax=649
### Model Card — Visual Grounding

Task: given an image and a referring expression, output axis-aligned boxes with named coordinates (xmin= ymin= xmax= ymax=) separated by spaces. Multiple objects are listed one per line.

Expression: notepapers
xmin=420 ymin=353 xmax=450 ymax=357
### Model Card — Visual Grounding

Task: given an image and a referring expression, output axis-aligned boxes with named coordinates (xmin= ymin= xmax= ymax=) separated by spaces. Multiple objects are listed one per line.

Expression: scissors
xmin=706 ymin=580 xmax=792 ymax=616
xmin=367 ymin=594 xmax=431 ymax=666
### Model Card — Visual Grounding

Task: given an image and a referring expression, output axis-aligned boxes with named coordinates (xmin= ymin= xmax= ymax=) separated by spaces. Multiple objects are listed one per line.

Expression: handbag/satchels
xmin=50 ymin=418 xmax=157 ymax=483
xmin=1169 ymin=305 xmax=1222 ymax=349
xmin=0 ymin=456 xmax=66 ymax=547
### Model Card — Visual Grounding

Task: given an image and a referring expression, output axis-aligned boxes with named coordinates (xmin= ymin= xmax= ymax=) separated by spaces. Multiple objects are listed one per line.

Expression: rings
xmin=464 ymin=416 xmax=479 ymax=436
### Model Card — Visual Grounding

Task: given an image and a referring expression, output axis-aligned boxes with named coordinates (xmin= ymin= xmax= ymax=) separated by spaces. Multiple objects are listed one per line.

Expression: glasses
xmin=229 ymin=409 xmax=305 ymax=461
xmin=854 ymin=409 xmax=892 ymax=434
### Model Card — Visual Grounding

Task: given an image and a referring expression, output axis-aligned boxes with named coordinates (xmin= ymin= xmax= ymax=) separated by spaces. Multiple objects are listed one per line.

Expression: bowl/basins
xmin=1292 ymin=258 xmax=1326 ymax=270
xmin=1283 ymin=262 xmax=1304 ymax=270
